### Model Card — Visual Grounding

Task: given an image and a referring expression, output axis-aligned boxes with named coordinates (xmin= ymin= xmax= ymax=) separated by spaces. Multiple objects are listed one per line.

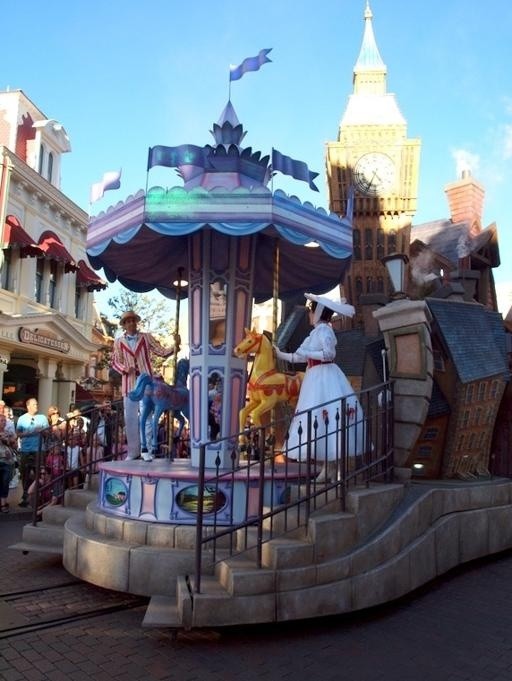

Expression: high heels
xmin=311 ymin=465 xmax=341 ymax=487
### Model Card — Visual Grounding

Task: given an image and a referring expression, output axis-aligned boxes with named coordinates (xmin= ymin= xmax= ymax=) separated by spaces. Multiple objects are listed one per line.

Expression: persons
xmin=111 ymin=312 xmax=181 ymax=462
xmin=272 ymin=293 xmax=374 ymax=483
xmin=0 ymin=398 xmax=190 ymax=516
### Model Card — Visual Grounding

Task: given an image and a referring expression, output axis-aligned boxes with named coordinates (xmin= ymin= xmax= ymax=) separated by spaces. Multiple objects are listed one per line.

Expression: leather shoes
xmin=125 ymin=451 xmax=152 ymax=461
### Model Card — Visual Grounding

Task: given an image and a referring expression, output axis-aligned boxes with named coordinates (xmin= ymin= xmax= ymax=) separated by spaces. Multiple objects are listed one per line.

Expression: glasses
xmin=39 ymin=473 xmax=46 ymax=475
xmin=31 ymin=417 xmax=34 ymax=426
xmin=51 ymin=411 xmax=60 ymax=415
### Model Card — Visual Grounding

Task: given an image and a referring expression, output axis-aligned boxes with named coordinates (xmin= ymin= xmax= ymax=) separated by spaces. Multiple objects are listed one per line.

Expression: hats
xmin=304 ymin=292 xmax=355 ymax=319
xmin=119 ymin=311 xmax=141 ymax=325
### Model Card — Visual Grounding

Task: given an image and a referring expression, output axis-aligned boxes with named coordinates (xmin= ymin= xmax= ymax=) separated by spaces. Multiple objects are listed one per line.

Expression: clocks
xmin=352 ymin=152 xmax=402 ymax=196
